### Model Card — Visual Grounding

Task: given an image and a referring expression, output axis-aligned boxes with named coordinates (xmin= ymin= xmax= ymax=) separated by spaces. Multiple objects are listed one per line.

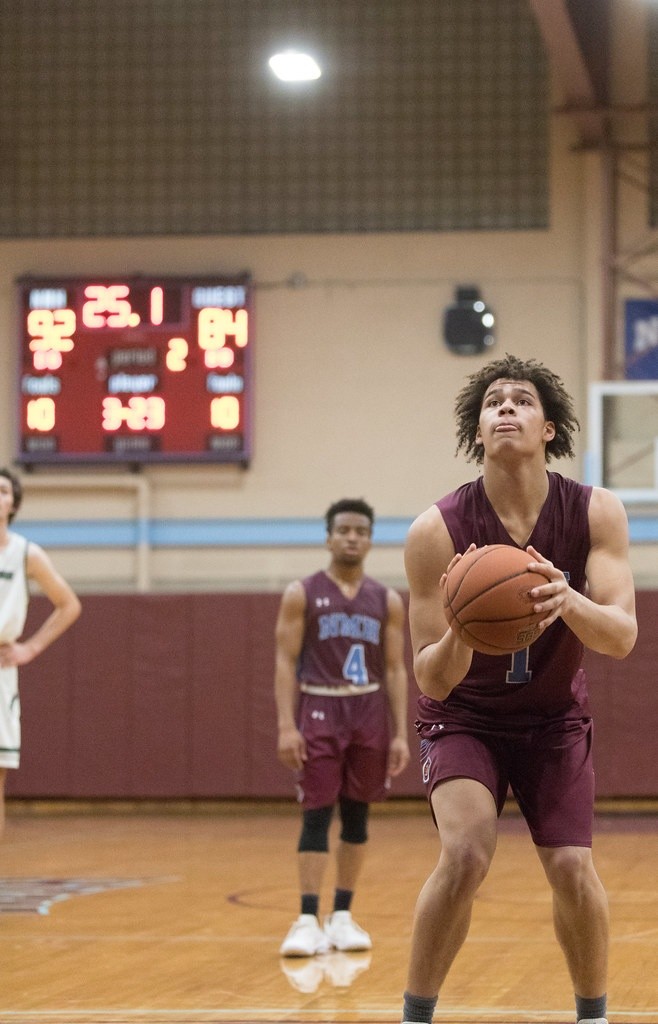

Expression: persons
xmin=401 ymin=356 xmax=638 ymax=1024
xmin=0 ymin=467 xmax=83 ymax=844
xmin=270 ymin=498 xmax=411 ymax=954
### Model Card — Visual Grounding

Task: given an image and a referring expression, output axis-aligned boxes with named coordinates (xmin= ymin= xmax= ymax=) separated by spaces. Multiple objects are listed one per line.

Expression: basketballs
xmin=441 ymin=541 xmax=552 ymax=657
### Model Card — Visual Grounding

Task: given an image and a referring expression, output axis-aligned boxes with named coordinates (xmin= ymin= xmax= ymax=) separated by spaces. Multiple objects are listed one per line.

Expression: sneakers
xmin=324 ymin=909 xmax=371 ymax=952
xmin=281 ymin=914 xmax=327 ymax=956
